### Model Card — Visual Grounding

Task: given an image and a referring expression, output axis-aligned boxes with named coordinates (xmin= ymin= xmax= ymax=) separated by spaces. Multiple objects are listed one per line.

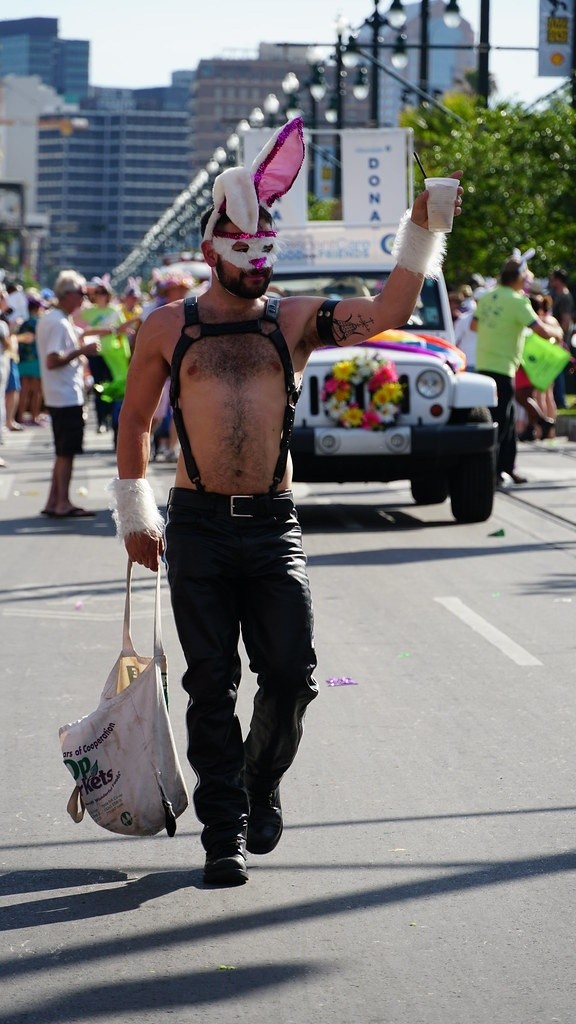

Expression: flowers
xmin=322 ymin=355 xmax=406 ymax=432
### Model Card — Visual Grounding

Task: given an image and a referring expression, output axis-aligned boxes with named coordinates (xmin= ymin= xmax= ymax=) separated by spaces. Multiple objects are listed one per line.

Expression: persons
xmin=110 ymin=167 xmax=466 ymax=885
xmin=447 ymin=248 xmax=576 ymax=484
xmin=0 ymin=251 xmax=206 ymax=517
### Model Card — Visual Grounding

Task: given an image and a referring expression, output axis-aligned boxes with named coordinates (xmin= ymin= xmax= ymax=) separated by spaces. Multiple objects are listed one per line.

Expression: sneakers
xmin=244 ymin=786 xmax=284 ymax=854
xmin=200 ymin=827 xmax=250 ymax=886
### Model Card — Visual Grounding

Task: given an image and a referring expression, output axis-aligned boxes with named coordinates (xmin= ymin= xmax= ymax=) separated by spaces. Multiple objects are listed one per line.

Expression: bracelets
xmin=110 ymin=477 xmax=162 ymax=539
xmin=389 ymin=209 xmax=446 ymax=281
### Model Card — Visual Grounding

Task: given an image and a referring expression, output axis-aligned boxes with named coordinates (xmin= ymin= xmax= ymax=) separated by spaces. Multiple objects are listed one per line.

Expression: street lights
xmin=110 ymin=0 xmax=491 ymax=286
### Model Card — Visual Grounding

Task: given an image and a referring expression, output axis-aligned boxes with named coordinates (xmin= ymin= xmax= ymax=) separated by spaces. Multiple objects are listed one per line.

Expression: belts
xmin=179 ymin=493 xmax=294 ymax=523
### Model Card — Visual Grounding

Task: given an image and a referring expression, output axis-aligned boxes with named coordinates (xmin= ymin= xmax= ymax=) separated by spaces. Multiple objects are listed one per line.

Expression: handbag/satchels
xmin=58 ymin=553 xmax=189 ymax=838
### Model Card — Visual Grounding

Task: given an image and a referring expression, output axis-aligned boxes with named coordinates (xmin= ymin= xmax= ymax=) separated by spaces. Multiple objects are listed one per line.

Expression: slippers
xmin=40 ymin=505 xmax=96 ymax=518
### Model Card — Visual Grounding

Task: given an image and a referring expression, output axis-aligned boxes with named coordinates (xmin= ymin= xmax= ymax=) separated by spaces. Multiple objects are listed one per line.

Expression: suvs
xmin=265 ymin=223 xmax=499 ymax=522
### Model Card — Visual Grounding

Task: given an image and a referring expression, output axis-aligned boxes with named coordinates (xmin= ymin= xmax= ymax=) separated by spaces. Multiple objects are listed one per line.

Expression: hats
xmin=52 ymin=270 xmax=88 ymax=295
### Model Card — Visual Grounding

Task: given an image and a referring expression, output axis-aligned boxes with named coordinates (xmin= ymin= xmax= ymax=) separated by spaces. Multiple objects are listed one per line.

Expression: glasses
xmin=69 ymin=290 xmax=89 ymax=300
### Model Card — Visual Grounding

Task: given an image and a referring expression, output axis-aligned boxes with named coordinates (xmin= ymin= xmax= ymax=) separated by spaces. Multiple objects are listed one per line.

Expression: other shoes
xmin=498 ymin=469 xmax=528 ymax=483
xmin=537 ymin=417 xmax=557 ymax=440
xmin=517 ymin=426 xmax=536 ymax=442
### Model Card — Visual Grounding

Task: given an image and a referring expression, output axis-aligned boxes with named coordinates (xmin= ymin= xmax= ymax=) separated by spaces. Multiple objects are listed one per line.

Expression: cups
xmin=83 ymin=335 xmax=101 ymax=351
xmin=424 ymin=177 xmax=460 ymax=233
